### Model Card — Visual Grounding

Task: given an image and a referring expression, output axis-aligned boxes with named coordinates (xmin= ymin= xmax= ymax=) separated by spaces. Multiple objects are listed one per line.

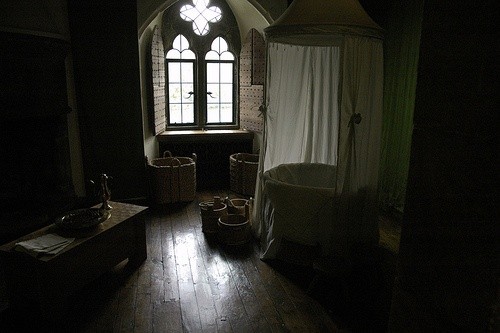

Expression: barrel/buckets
xmin=199 ymin=196 xmax=228 ymax=234
xmin=216 ymin=204 xmax=251 ymax=247
xmin=224 ymin=196 xmax=253 ymax=219
xmin=229 ymin=153 xmax=259 ymax=196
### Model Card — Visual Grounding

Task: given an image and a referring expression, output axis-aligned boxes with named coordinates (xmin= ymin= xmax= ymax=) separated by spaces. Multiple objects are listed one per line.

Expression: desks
xmin=2 ymin=200 xmax=149 ymax=311
